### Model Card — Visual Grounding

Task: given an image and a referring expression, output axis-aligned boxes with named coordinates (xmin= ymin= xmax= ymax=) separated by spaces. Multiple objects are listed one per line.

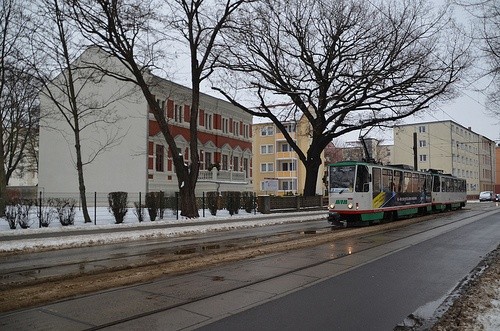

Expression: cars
xmin=494 ymin=194 xmax=500 ymax=201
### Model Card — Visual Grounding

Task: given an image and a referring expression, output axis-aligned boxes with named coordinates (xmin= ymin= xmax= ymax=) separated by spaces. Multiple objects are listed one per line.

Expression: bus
xmin=327 ymin=120 xmax=467 ymax=227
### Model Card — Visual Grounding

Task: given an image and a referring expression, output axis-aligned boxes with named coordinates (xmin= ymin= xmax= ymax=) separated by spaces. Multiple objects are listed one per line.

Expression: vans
xmin=479 ymin=191 xmax=493 ymax=202
